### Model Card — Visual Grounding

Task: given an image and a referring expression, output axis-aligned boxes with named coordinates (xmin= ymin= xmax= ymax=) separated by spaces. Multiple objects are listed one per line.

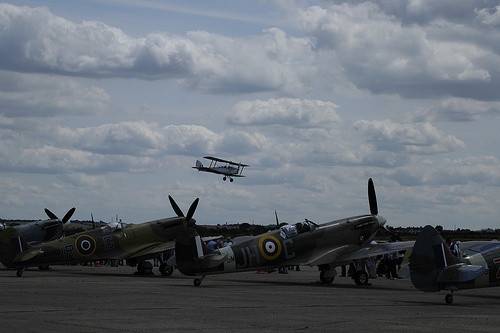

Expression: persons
xmin=80 ymin=260 xmax=118 ymax=268
xmin=338 ymin=239 xmax=406 ymax=280
xmin=207 ymin=235 xmax=233 ymax=250
xmin=274 ymin=265 xmax=301 ymax=274
xmin=449 ymin=239 xmax=461 ymax=258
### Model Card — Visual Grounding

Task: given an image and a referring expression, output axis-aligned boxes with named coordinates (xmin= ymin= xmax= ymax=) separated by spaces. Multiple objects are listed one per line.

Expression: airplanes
xmin=191 ymin=155 xmax=250 ymax=182
xmin=0 ymin=194 xmax=222 ymax=277
xmin=396 ymin=225 xmax=500 ymax=303
xmin=192 ymin=178 xmax=415 ymax=287
